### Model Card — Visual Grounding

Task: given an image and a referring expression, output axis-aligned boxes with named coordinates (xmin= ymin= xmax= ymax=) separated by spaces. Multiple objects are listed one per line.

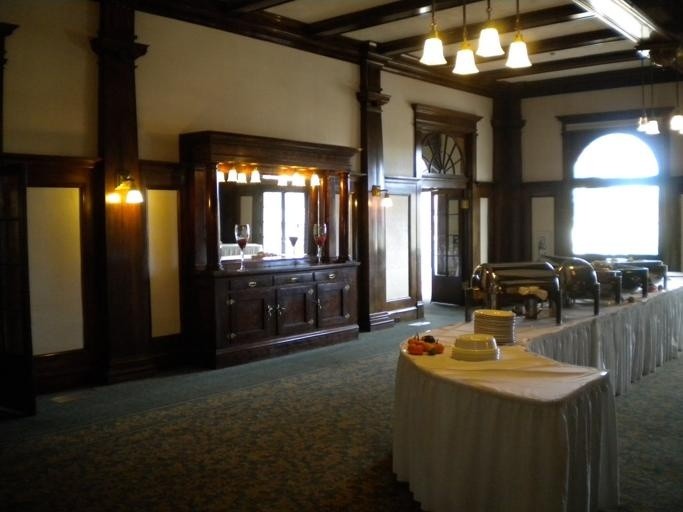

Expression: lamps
xmin=216 ymin=160 xmax=323 ymax=188
xmin=105 ymin=151 xmax=146 ymax=208
xmin=571 ymin=1 xmax=683 ymax=138
xmin=418 ymin=0 xmax=533 ymax=77
xmin=371 ymin=185 xmax=390 ymax=200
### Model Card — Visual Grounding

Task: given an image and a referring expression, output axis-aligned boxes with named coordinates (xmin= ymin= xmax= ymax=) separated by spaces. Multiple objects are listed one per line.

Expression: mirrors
xmin=218 ymin=161 xmax=330 ymax=268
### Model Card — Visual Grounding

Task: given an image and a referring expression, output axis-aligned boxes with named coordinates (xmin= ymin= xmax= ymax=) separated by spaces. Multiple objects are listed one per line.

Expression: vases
xmin=519 ymin=296 xmax=542 ymax=319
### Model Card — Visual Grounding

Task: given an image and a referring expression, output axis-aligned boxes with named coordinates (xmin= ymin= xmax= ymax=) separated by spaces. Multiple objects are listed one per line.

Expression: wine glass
xmin=313 ymin=223 xmax=327 ymax=266
xmin=234 ymin=223 xmax=250 ymax=272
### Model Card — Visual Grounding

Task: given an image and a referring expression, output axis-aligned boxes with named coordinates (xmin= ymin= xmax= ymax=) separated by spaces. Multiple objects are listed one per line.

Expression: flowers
xmin=518 ymin=286 xmax=549 ymax=301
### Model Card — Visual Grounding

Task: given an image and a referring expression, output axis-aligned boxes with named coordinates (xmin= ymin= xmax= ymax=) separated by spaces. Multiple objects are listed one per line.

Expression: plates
xmin=473 ymin=309 xmax=517 ymax=347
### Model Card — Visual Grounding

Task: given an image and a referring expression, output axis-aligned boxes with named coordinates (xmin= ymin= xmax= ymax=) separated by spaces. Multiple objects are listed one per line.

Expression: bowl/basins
xmin=450 ymin=333 xmax=501 ymax=362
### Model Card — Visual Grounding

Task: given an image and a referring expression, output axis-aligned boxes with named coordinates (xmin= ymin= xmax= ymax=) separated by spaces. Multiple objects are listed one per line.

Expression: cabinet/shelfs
xmin=178 ymin=264 xmax=360 ymax=371
